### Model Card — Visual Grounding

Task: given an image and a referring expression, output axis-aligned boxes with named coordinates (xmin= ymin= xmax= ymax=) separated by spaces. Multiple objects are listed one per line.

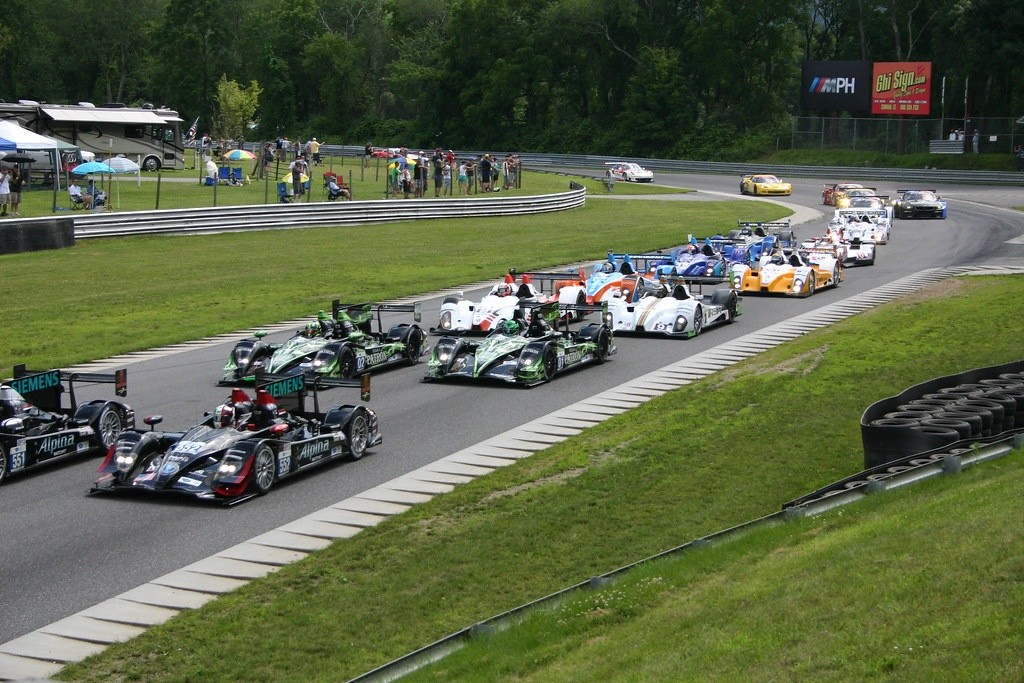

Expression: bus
xmin=0 ymin=99 xmax=189 ymax=173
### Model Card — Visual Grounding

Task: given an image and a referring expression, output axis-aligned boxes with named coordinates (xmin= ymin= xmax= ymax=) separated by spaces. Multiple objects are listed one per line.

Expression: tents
xmin=0 ymin=136 xmax=18 ymax=169
xmin=17 ymin=135 xmax=90 ymax=191
xmin=1 ymin=119 xmax=60 ymax=213
xmin=80 ymin=151 xmax=95 ymax=180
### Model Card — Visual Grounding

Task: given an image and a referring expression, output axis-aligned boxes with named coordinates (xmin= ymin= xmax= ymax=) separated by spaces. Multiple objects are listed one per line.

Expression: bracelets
xmin=16 ymin=177 xmax=18 ymax=179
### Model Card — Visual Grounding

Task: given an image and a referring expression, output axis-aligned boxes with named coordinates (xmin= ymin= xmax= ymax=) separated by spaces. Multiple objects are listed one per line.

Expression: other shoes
xmin=10 ymin=212 xmax=16 ymax=217
xmin=14 ymin=212 xmax=20 ymax=216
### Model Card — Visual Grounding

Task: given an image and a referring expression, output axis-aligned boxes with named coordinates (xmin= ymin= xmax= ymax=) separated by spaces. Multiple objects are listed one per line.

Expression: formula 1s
xmin=0 ymin=183 xmax=949 ymax=509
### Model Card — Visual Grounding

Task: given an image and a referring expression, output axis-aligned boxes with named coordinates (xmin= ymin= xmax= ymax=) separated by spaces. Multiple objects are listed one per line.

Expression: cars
xmin=605 ymin=162 xmax=654 ymax=183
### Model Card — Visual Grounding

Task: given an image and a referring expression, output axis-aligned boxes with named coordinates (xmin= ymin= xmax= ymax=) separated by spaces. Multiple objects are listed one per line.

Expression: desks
xmin=206 ymin=176 xmax=218 ymax=186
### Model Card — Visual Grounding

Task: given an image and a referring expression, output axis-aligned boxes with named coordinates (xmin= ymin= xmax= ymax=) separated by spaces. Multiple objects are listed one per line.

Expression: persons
xmin=742 ymin=227 xmax=752 ymax=237
xmin=70 ymin=179 xmax=102 ymax=209
xmin=0 ymin=166 xmax=25 ymax=216
xmin=603 ymin=262 xmax=613 ymax=274
xmin=497 ymin=284 xmax=510 ymax=297
xmin=212 ymin=405 xmax=234 ymax=429
xmin=203 ymin=134 xmax=521 ymax=201
xmin=502 ymin=320 xmax=520 ymax=337
xmin=305 ymin=321 xmax=323 ymax=340
xmin=686 ymin=245 xmax=697 ymax=255
xmin=946 ymin=127 xmax=1024 ymax=172
xmin=770 ymin=253 xmax=782 ymax=265
xmin=652 ymin=283 xmax=668 ymax=297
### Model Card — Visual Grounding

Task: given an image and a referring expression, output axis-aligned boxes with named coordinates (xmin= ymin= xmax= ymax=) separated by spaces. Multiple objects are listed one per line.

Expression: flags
xmin=189 ymin=124 xmax=197 ymax=146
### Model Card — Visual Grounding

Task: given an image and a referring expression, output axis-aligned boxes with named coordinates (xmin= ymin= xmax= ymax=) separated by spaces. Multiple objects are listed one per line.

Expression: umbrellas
xmin=1 ymin=153 xmax=36 ymax=165
xmin=71 ymin=160 xmax=115 ymax=211
xmin=102 ymin=157 xmax=141 ymax=209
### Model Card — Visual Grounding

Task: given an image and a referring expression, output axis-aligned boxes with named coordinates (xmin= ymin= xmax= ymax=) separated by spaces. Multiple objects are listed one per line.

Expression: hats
xmin=330 ymin=176 xmax=336 ymax=181
xmin=419 ymin=151 xmax=424 ymax=153
xmin=449 ymin=150 xmax=452 ymax=153
xmin=469 ymin=157 xmax=476 ymax=161
xmin=295 ymin=160 xmax=303 ymax=165
xmin=313 ymin=138 xmax=316 ymax=140
xmin=485 ymin=154 xmax=489 ymax=158
xmin=89 ymin=180 xmax=96 ymax=185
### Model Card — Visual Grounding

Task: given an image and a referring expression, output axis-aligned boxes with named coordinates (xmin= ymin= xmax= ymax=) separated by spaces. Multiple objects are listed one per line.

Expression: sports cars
xmin=738 ymin=173 xmax=792 ymax=196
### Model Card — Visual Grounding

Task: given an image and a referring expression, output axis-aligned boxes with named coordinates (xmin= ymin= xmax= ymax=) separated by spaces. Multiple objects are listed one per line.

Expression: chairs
xmin=0 ymin=212 xmax=879 ymax=442
xmin=277 ymin=182 xmax=293 ymax=203
xmin=337 ymin=175 xmax=349 ymax=191
xmin=218 ymin=167 xmax=233 ymax=185
xmin=323 ymin=171 xmax=336 ymax=196
xmin=69 ymin=194 xmax=83 ymax=210
xmin=233 ymin=166 xmax=243 ymax=186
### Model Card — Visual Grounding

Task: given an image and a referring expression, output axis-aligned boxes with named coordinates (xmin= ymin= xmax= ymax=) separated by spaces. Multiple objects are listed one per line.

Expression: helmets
xmin=822 ymin=235 xmax=832 ymax=244
xmin=213 ymin=405 xmax=232 ymax=428
xmin=848 ymin=215 xmax=859 ymax=224
xmin=686 ymin=245 xmax=697 ymax=255
xmin=601 ymin=263 xmax=613 ymax=273
xmin=503 ymin=319 xmax=519 ymax=337
xmin=305 ymin=321 xmax=322 ymax=338
xmin=652 ymin=283 xmax=665 ymax=298
xmin=771 ymin=253 xmax=783 ymax=265
xmin=497 ymin=283 xmax=511 ymax=297
xmin=742 ymin=227 xmax=752 ymax=237
